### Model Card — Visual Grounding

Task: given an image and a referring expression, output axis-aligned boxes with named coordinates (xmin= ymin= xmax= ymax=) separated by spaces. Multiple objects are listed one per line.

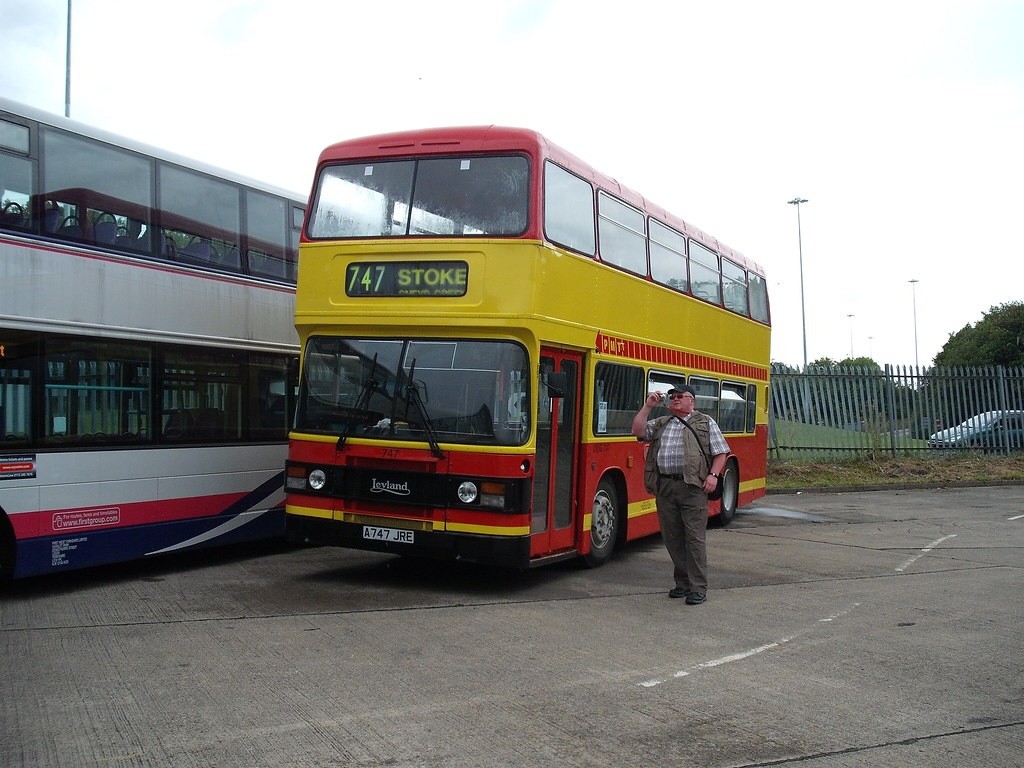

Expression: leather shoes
xmin=669 ymin=587 xmax=690 ymax=598
xmin=685 ymin=592 xmax=707 ymax=604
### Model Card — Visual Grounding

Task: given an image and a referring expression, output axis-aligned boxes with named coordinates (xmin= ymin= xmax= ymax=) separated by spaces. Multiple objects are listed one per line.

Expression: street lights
xmin=789 ymin=198 xmax=812 ymax=425
xmin=908 ymin=280 xmax=921 ymax=366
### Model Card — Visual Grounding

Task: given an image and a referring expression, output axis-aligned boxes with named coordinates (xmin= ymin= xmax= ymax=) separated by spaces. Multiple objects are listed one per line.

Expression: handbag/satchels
xmin=708 ymin=475 xmax=723 ymax=500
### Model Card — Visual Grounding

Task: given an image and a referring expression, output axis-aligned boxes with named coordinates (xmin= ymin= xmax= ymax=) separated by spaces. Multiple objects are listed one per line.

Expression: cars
xmin=927 ymin=410 xmax=1024 ymax=451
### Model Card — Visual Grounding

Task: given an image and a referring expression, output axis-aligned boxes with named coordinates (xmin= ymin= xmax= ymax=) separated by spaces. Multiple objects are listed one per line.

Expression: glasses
xmin=669 ymin=395 xmax=694 ymax=400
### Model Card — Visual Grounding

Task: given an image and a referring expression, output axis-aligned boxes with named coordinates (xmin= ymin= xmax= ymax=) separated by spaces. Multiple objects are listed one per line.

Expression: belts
xmin=660 ymin=474 xmax=683 ymax=480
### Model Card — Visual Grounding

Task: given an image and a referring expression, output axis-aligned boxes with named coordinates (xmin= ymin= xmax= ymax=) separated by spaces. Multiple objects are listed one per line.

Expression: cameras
xmin=657 ymin=394 xmax=668 ymax=401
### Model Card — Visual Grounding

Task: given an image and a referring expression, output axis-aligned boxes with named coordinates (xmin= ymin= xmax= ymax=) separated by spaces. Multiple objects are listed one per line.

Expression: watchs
xmin=708 ymin=472 xmax=719 ymax=479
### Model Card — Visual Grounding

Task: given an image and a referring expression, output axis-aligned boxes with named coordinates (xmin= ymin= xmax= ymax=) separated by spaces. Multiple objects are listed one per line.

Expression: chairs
xmin=4 ymin=199 xmax=288 ymax=285
xmin=648 ymin=393 xmax=753 ymax=431
xmin=667 ymin=279 xmax=744 ymax=313
xmin=6 ymin=392 xmax=297 ymax=446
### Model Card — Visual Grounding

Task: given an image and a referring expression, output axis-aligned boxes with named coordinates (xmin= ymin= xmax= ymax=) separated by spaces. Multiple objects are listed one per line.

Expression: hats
xmin=668 ymin=385 xmax=695 ymax=398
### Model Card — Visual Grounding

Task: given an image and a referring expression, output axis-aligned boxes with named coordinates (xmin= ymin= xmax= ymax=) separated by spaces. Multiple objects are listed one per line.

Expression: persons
xmin=632 ymin=384 xmax=732 ymax=603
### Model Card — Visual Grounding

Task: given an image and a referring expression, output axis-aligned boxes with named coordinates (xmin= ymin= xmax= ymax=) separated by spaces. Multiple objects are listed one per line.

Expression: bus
xmin=0 ymin=94 xmax=437 ymax=596
xmin=282 ymin=123 xmax=773 ymax=570
